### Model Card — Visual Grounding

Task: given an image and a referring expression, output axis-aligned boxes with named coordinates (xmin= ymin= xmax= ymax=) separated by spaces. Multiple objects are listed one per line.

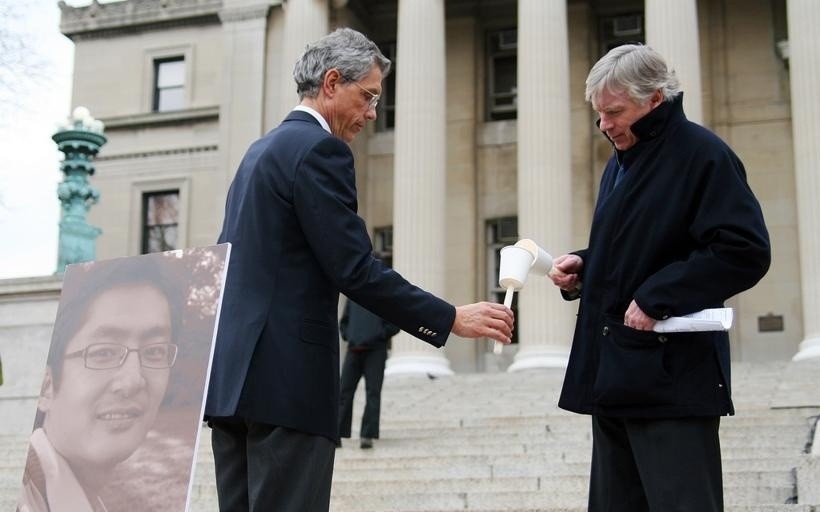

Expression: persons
xmin=550 ymin=46 xmax=771 ymax=512
xmin=17 ymin=254 xmax=186 ymax=512
xmin=204 ymin=26 xmax=514 ymax=512
xmin=337 ymin=249 xmax=401 ymax=449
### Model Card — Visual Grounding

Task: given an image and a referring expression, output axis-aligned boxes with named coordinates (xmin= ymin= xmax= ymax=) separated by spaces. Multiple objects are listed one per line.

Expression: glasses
xmin=62 ymin=342 xmax=179 ymax=368
xmin=342 ymin=73 xmax=379 ymax=109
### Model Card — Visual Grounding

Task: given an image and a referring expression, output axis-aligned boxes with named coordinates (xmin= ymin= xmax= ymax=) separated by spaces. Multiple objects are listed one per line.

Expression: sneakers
xmin=337 ymin=435 xmax=373 ymax=448
